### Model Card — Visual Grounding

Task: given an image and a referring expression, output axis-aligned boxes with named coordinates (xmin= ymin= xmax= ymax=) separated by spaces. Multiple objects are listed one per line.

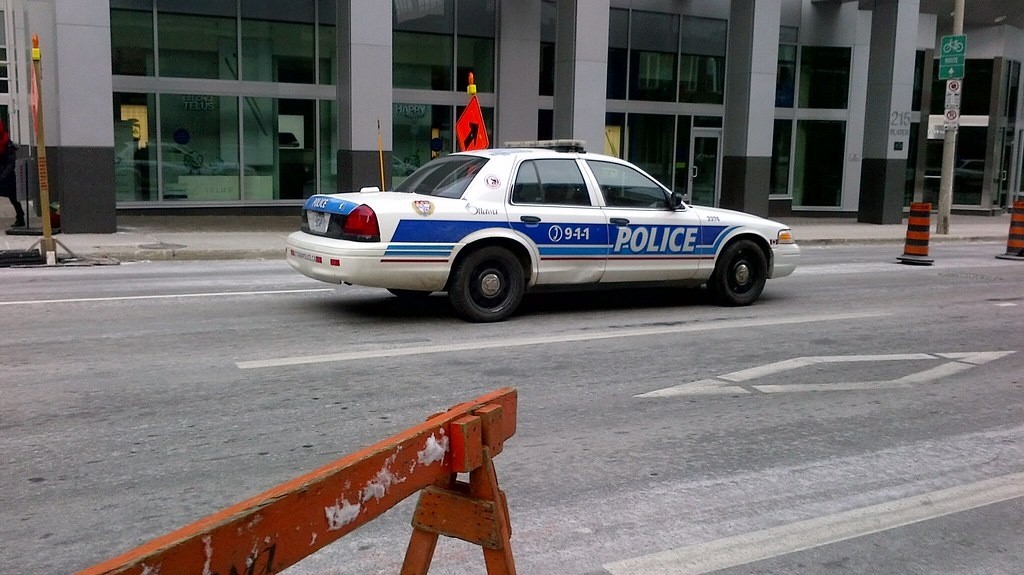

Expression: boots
xmin=10 ymin=202 xmax=25 ymax=227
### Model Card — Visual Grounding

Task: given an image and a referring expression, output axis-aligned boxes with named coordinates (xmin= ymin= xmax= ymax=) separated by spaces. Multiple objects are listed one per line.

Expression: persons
xmin=0 ymin=115 xmax=26 ymax=227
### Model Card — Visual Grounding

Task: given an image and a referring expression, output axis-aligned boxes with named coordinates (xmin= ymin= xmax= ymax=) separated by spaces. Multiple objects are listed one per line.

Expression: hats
xmin=0 ymin=120 xmax=4 ymax=132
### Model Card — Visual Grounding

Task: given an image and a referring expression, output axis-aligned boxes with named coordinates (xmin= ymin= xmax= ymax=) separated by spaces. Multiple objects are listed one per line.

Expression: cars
xmin=926 ymin=157 xmax=986 ymax=192
xmin=114 ymin=141 xmax=261 ymax=194
xmin=284 ymin=139 xmax=803 ymax=323
xmin=392 ymin=154 xmax=419 ymax=176
